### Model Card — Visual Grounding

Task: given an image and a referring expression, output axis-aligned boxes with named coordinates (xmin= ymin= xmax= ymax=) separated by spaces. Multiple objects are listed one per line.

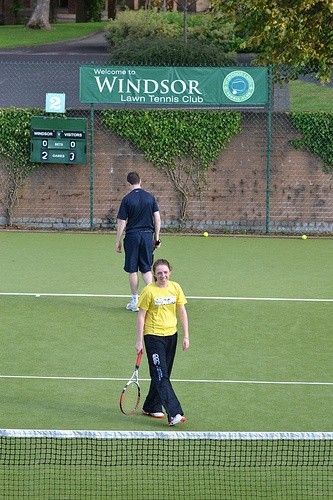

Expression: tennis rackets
xmin=151 ymin=239 xmax=161 ymax=265
xmin=120 ymin=351 xmax=142 ymax=416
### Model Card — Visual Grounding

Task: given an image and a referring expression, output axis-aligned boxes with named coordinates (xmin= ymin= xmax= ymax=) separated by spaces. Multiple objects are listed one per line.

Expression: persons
xmin=135 ymin=258 xmax=190 ymax=427
xmin=116 ymin=172 xmax=162 ymax=312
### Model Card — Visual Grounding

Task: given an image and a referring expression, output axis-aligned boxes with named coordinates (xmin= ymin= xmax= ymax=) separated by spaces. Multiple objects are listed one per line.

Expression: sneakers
xmin=141 ymin=408 xmax=165 ymax=418
xmin=126 ymin=302 xmax=139 ymax=312
xmin=168 ymin=413 xmax=186 ymax=426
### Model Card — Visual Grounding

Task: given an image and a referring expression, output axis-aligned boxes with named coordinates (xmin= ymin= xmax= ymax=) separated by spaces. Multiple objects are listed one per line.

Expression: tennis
xmin=203 ymin=232 xmax=208 ymax=237
xmin=302 ymin=235 xmax=307 ymax=239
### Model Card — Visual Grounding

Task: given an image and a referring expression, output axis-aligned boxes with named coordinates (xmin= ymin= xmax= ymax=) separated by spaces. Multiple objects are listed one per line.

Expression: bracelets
xmin=184 ymin=336 xmax=189 ymax=341
